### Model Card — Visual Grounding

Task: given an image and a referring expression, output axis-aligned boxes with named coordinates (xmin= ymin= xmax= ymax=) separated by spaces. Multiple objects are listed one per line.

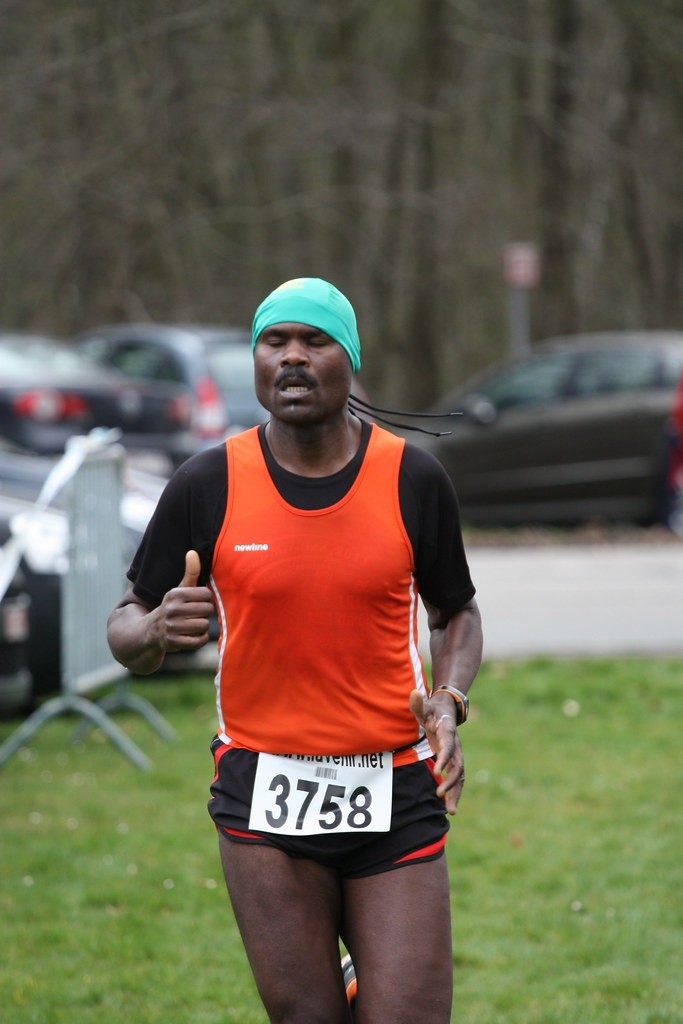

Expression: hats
xmin=252 ymin=277 xmax=360 ymax=376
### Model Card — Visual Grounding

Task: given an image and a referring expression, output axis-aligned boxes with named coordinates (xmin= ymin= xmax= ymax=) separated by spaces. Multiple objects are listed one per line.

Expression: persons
xmin=106 ymin=278 xmax=484 ymax=1024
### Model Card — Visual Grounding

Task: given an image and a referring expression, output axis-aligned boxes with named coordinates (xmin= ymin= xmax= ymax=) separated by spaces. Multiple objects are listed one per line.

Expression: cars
xmin=404 ymin=330 xmax=683 ymax=526
xmin=0 ymin=322 xmax=380 ymax=711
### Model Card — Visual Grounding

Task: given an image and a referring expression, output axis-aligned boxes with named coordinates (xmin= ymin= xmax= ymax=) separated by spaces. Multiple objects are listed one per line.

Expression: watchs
xmin=430 ymin=685 xmax=470 ymax=727
